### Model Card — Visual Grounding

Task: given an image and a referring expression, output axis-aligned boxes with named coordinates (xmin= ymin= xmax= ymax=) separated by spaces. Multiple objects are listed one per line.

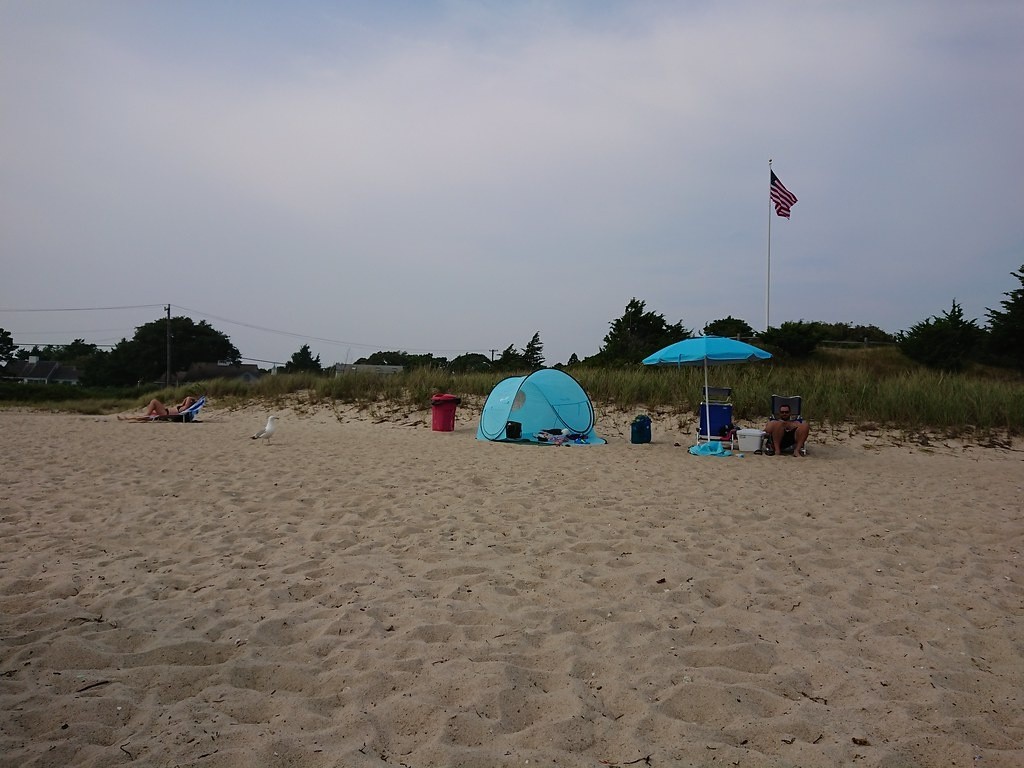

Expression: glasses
xmin=781 ymin=411 xmax=790 ymax=414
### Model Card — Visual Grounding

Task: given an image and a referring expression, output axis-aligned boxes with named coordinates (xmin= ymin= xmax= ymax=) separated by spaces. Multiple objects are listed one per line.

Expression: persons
xmin=118 ymin=397 xmax=197 ymax=421
xmin=766 ymin=404 xmax=809 ymax=458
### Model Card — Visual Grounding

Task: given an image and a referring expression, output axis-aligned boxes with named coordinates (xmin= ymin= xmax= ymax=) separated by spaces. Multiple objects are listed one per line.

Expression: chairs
xmin=770 ymin=394 xmax=807 ymax=456
xmin=149 ymin=396 xmax=206 ymax=423
xmin=697 ymin=402 xmax=734 ymax=451
xmin=701 ymin=384 xmax=731 ymax=402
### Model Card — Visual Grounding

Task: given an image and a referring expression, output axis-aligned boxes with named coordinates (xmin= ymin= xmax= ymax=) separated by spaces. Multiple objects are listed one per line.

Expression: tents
xmin=476 ymin=368 xmax=607 ymax=446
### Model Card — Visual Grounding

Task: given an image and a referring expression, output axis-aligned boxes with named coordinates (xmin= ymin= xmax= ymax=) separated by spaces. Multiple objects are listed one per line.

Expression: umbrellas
xmin=642 ymin=334 xmax=773 ymax=442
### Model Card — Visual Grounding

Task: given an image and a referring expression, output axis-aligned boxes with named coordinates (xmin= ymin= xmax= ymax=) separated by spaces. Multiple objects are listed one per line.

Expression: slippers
xmin=754 ymin=450 xmax=762 ymax=455
xmin=765 ymin=450 xmax=772 ymax=456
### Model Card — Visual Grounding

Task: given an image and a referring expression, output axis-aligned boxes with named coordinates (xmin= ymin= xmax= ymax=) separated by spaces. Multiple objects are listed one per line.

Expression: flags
xmin=771 ymin=168 xmax=798 ymax=220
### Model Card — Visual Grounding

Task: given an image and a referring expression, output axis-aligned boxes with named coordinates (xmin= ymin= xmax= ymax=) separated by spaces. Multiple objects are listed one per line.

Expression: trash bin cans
xmin=431 ymin=392 xmax=462 ymax=433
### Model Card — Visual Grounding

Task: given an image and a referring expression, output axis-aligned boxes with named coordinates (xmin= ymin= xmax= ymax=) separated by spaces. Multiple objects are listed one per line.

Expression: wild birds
xmin=250 ymin=416 xmax=280 ymax=444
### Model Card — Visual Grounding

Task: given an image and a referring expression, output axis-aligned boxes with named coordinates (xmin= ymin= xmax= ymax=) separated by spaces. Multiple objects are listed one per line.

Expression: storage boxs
xmin=735 ymin=429 xmax=766 ymax=452
